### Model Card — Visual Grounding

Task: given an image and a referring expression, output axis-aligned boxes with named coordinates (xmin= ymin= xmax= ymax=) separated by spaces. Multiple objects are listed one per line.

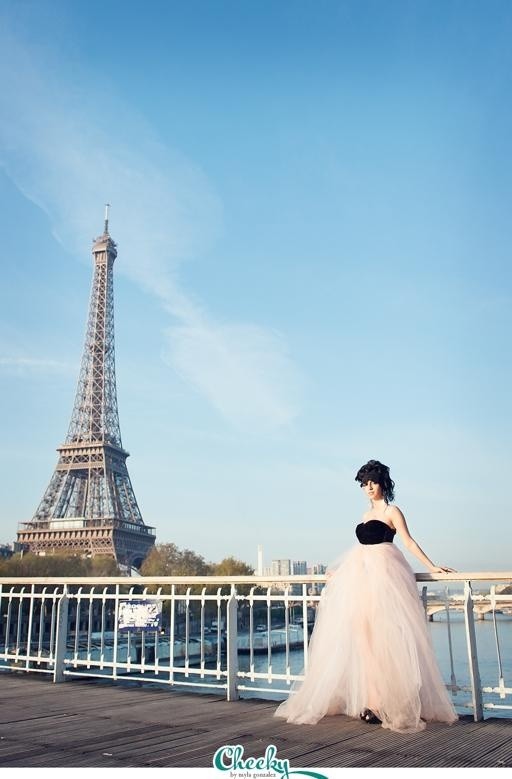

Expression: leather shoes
xmin=360 ymin=709 xmax=381 ymax=723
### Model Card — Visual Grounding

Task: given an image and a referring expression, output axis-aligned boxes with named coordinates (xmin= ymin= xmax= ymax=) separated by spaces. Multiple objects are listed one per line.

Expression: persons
xmin=274 ymin=460 xmax=459 ymax=733
